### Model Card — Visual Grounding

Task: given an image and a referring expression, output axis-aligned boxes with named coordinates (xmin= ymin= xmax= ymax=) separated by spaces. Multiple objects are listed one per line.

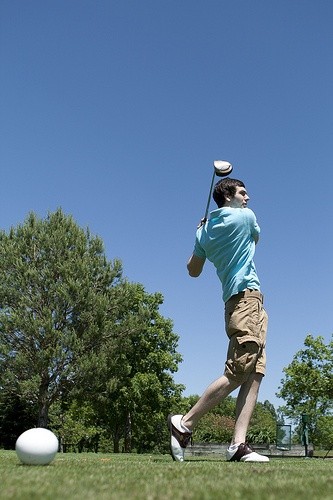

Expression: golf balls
xmin=15 ymin=428 xmax=58 ymax=465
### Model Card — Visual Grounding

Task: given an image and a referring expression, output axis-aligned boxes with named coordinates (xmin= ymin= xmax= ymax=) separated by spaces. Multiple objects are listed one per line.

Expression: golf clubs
xmin=203 ymin=161 xmax=233 ymax=223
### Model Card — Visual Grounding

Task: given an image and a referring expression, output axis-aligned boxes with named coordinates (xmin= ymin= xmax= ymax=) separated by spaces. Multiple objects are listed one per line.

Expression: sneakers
xmin=167 ymin=413 xmax=195 ymax=462
xmin=226 ymin=440 xmax=271 ymax=462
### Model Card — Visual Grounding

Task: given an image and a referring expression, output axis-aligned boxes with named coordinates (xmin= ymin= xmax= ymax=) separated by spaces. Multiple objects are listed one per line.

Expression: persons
xmin=168 ymin=177 xmax=270 ymax=463
